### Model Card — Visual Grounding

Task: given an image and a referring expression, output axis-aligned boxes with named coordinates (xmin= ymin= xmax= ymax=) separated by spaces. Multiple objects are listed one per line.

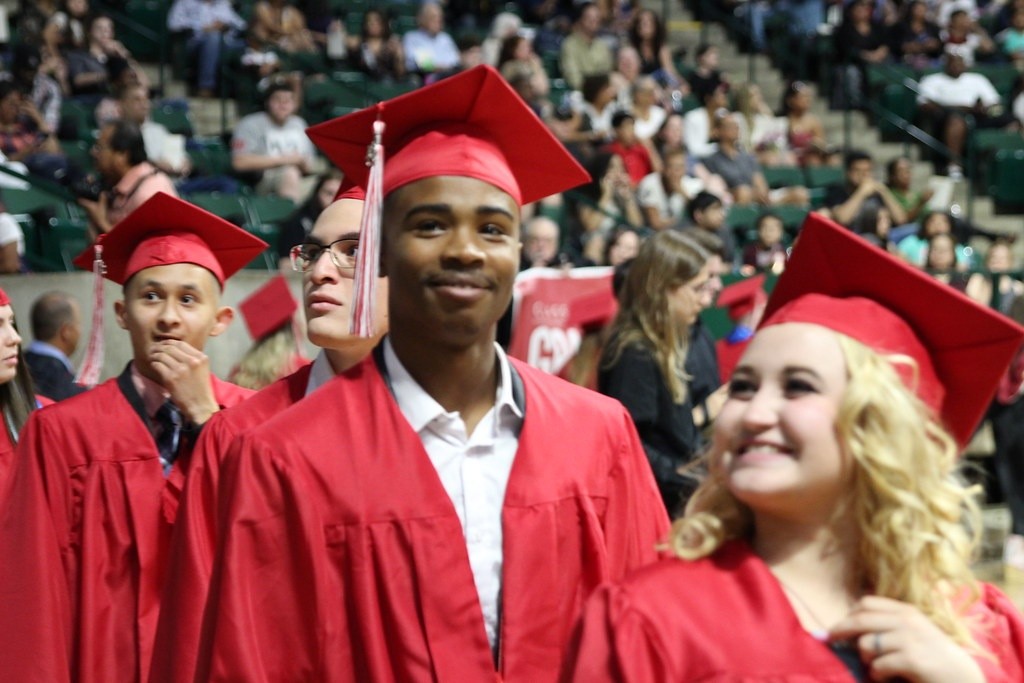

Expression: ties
xmin=156 ymin=400 xmax=183 ymax=470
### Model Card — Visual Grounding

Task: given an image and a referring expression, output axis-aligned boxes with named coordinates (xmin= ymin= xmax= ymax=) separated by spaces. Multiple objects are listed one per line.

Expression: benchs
xmin=0 ymin=0 xmax=1023 ymax=513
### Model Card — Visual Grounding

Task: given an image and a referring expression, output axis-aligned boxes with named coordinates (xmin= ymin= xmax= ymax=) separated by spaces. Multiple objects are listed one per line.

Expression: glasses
xmin=289 ymin=236 xmax=360 ymax=273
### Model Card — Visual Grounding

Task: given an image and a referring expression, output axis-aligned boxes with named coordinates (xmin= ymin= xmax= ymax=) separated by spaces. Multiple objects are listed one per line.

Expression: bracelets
xmin=700 ymin=400 xmax=709 ymax=423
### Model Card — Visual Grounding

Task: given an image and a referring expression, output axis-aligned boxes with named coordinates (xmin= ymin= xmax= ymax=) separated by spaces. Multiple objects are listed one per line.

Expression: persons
xmin=613 ymin=259 xmax=721 ymax=408
xmin=597 ymin=229 xmax=734 ymax=523
xmin=22 ymin=291 xmax=95 ymax=402
xmin=0 ymin=288 xmax=59 ymax=482
xmin=572 ymin=212 xmax=1024 ymax=682
xmin=165 ymin=178 xmax=391 ymax=682
xmin=207 ymin=64 xmax=672 ymax=683
xmin=1 ymin=190 xmax=263 ymax=683
xmin=0 ymin=0 xmax=1024 ymax=409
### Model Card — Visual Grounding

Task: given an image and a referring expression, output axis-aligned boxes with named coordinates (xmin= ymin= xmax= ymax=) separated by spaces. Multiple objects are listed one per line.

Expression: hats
xmin=1 ymin=288 xmax=10 ymax=305
xmin=72 ymin=191 xmax=270 ymax=386
xmin=563 ymin=283 xmax=616 ymax=331
xmin=304 ymin=62 xmax=591 ymax=337
xmin=238 ymin=273 xmax=298 ymax=340
xmin=717 ymin=271 xmax=766 ymax=317
xmin=335 ymin=171 xmax=367 ymax=201
xmin=751 ymin=210 xmax=1024 ymax=455
